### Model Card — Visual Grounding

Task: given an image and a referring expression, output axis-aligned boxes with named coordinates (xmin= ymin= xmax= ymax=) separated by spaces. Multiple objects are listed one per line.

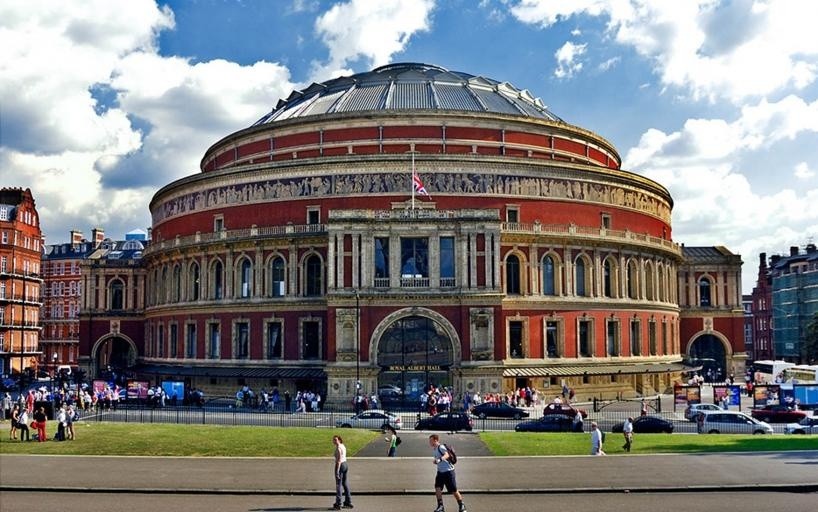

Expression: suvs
xmin=413 ymin=412 xmax=473 ymax=431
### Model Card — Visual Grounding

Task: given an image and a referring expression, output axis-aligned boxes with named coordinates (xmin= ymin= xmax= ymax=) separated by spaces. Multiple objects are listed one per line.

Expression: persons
xmin=621 ymin=416 xmax=635 ymax=453
xmin=236 ymin=385 xmax=322 ymax=413
xmin=689 ymin=365 xmax=754 ymax=398
xmin=427 ymin=385 xmax=574 ymax=416
xmin=353 ymin=393 xmax=377 ymax=413
xmin=589 ymin=421 xmax=605 ymax=454
xmin=429 ymin=433 xmax=467 ymax=511
xmin=385 ymin=426 xmax=398 ymax=456
xmin=573 ymin=407 xmax=585 ymax=432
xmin=639 ymin=396 xmax=648 ymax=417
xmin=328 ymin=434 xmax=354 ymax=511
xmin=0 ymin=380 xmax=205 ymax=444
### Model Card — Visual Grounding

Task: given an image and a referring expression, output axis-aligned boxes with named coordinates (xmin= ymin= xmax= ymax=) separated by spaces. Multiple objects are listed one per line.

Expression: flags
xmin=411 ymin=150 xmax=433 ymax=201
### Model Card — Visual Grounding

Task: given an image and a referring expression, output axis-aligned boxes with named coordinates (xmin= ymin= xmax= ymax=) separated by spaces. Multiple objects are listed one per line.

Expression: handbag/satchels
xmin=15 ymin=422 xmax=22 ymax=429
xmin=30 ymin=421 xmax=37 ymax=429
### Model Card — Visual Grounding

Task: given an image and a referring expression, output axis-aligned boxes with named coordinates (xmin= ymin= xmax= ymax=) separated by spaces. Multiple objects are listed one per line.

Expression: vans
xmin=59 ymin=365 xmax=78 ymax=375
xmin=695 ymin=409 xmax=774 ymax=436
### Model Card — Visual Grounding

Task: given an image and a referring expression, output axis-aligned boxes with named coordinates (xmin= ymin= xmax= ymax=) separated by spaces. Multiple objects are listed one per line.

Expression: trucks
xmin=776 ymin=365 xmax=818 ymax=384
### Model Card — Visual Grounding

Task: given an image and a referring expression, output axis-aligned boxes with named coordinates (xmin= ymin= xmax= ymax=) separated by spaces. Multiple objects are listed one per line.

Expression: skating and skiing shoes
xmin=459 ymin=503 xmax=466 ymax=512
xmin=333 ymin=504 xmax=341 ymax=510
xmin=434 ymin=506 xmax=444 ymax=512
xmin=343 ymin=502 xmax=353 ymax=508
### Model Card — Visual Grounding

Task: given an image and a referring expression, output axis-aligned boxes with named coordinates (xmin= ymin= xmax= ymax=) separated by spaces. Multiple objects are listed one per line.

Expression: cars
xmin=379 ymin=385 xmax=402 ymax=398
xmin=782 ymin=416 xmax=818 ymax=435
xmin=515 ymin=414 xmax=585 ymax=433
xmin=203 ymin=398 xmax=249 ymax=409
xmin=128 ymin=388 xmax=140 ymax=397
xmin=117 ymin=389 xmax=126 ymax=400
xmin=751 ymin=406 xmax=805 ymax=425
xmin=685 ymin=404 xmax=726 ymax=422
xmin=26 ymin=367 xmax=49 ymax=378
xmin=334 ymin=410 xmax=404 ymax=430
xmin=543 ymin=402 xmax=587 ymax=419
xmin=469 ymin=402 xmax=529 ymax=420
xmin=2 ymin=379 xmax=20 ymax=391
xmin=612 ymin=415 xmax=673 ymax=435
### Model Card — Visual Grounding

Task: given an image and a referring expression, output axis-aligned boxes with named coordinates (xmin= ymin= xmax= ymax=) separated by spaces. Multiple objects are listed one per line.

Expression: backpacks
xmin=602 ymin=432 xmax=605 ymax=443
xmin=396 ymin=437 xmax=402 ymax=446
xmin=437 ymin=443 xmax=457 ymax=464
xmin=73 ymin=412 xmax=79 ymax=421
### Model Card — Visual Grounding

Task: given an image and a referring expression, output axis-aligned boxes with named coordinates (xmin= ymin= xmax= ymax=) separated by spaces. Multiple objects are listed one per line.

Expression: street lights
xmin=350 ymin=288 xmax=361 ymax=414
xmin=53 ymin=352 xmax=57 ymax=378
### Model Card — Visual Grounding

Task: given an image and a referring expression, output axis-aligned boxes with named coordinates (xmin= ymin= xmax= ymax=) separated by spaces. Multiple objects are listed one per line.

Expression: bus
xmin=754 ymin=359 xmax=794 ymax=384
